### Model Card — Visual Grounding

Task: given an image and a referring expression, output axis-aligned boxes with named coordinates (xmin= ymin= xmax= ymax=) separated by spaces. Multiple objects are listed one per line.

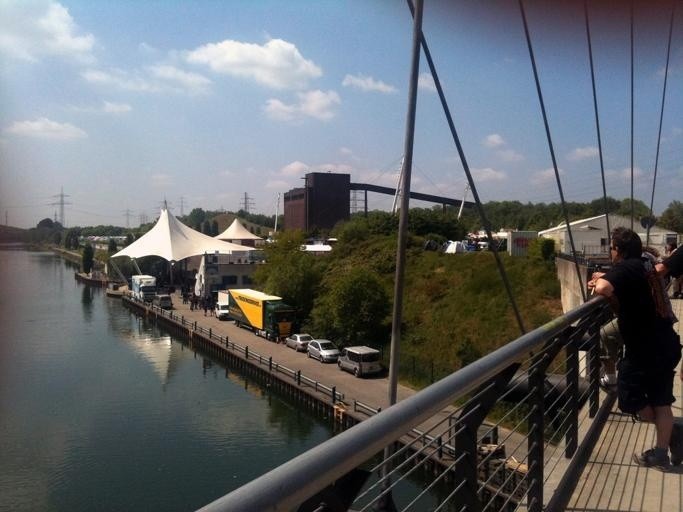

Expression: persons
xmin=180 ymin=283 xmax=214 ymax=316
xmin=585 ymin=224 xmax=683 ymax=468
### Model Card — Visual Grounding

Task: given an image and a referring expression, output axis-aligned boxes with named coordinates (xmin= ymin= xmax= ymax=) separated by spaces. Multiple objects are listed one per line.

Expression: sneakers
xmin=669 ymin=424 xmax=683 ymax=466
xmin=632 ymin=446 xmax=671 ymax=472
xmin=599 ymin=377 xmax=617 ymax=395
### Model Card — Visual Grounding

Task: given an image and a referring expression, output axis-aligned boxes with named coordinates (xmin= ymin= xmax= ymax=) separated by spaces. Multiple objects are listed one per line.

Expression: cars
xmin=306 ymin=339 xmax=342 ymax=363
xmin=285 ymin=334 xmax=314 ymax=351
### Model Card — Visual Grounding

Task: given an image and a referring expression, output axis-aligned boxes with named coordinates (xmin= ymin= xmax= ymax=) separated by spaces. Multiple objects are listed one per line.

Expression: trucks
xmin=131 ymin=274 xmax=157 ymax=301
xmin=227 ymin=289 xmax=296 ymax=343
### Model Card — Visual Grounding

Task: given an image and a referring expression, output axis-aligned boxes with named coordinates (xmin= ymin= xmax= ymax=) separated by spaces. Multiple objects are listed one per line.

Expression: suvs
xmin=151 ymin=294 xmax=172 ymax=310
xmin=213 ymin=301 xmax=229 ymax=321
xmin=337 ymin=345 xmax=382 ymax=377
